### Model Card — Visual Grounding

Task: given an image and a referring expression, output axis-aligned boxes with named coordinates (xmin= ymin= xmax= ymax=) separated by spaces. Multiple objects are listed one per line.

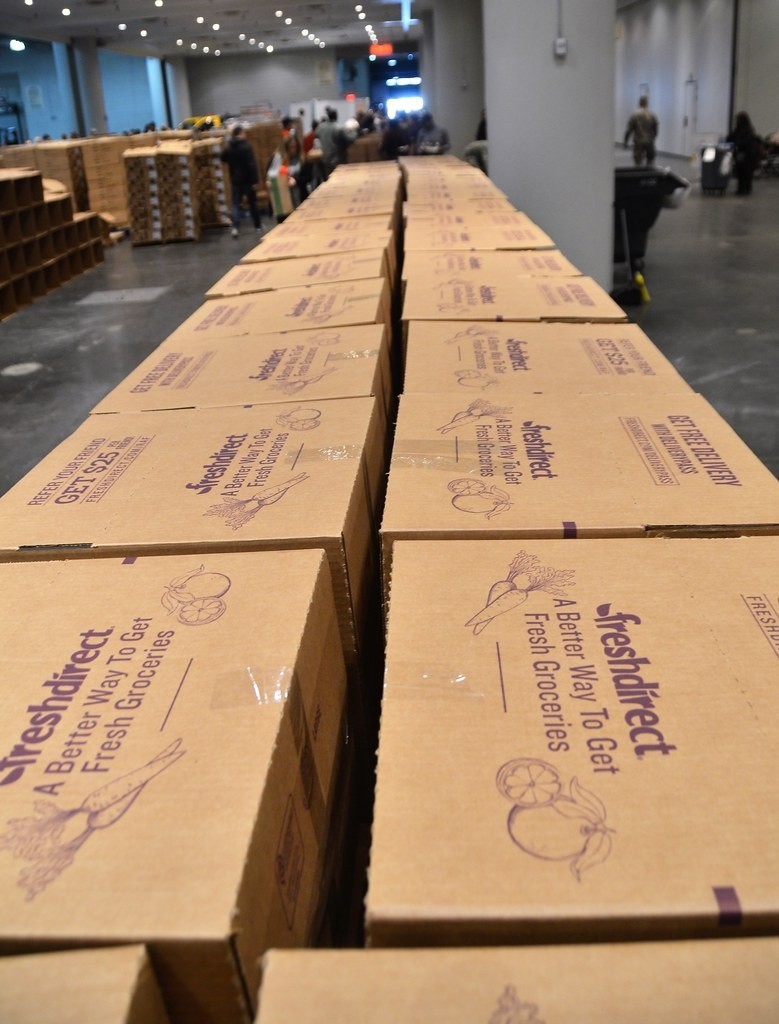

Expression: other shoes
xmin=232 ymin=228 xmax=240 ymax=236
xmin=257 ymin=227 xmax=264 ymax=232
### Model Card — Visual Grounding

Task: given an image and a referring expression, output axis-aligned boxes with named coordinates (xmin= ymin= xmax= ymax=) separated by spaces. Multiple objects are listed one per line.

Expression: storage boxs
xmin=0 ymin=120 xmax=779 ymax=1024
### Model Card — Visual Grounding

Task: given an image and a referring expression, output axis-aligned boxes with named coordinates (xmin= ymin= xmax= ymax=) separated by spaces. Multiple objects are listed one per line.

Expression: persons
xmin=25 ymin=121 xmax=170 ymax=145
xmin=718 ymin=111 xmax=760 ymax=194
xmin=622 ymin=96 xmax=660 ymax=167
xmin=265 ymin=105 xmax=453 ymax=223
xmin=474 ymin=108 xmax=487 ymax=141
xmin=219 ymin=127 xmax=266 ymax=238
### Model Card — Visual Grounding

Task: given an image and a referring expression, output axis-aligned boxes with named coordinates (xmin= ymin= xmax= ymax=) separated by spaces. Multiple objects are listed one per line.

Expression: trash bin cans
xmin=700 ymin=142 xmax=735 ymax=197
xmin=614 ymin=165 xmax=692 ymax=284
